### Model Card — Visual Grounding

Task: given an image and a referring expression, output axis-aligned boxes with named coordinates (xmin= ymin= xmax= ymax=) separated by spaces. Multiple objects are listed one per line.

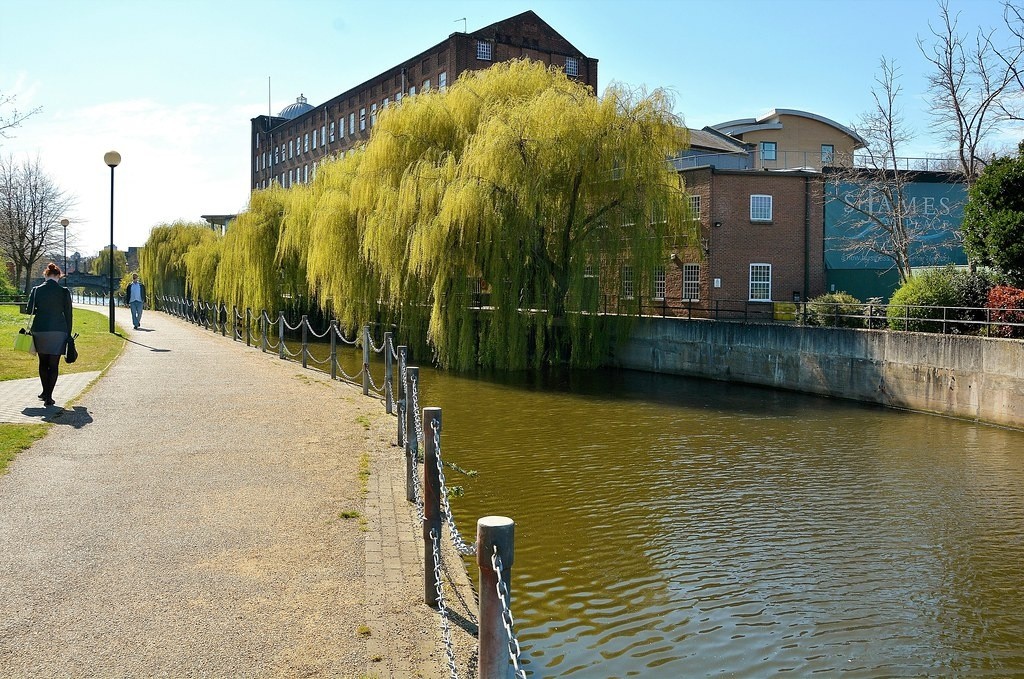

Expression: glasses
xmin=133 ymin=277 xmax=138 ymax=280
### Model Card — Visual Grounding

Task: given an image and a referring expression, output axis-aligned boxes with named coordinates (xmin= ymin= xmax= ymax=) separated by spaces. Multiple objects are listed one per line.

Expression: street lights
xmin=103 ymin=150 xmax=122 ymax=333
xmin=60 ymin=218 xmax=69 ymax=287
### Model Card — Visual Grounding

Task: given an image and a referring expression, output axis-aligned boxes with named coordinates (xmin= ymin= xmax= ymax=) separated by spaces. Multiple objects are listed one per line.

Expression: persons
xmin=26 ymin=263 xmax=73 ymax=406
xmin=126 ymin=273 xmax=146 ymax=329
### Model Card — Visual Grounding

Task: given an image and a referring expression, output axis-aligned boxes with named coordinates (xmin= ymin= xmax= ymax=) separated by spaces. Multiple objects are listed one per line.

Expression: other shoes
xmin=134 ymin=326 xmax=137 ymax=329
xmin=45 ymin=398 xmax=55 ymax=404
xmin=138 ymin=323 xmax=140 ymax=326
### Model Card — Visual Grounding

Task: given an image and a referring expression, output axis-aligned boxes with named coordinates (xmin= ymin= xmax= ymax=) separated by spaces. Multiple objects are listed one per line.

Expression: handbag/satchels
xmin=15 ymin=333 xmax=36 ymax=356
xmin=64 ymin=333 xmax=79 ymax=364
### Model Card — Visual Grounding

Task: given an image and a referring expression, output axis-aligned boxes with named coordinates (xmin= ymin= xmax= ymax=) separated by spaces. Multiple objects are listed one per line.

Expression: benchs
xmin=15 ymin=303 xmax=26 ymax=314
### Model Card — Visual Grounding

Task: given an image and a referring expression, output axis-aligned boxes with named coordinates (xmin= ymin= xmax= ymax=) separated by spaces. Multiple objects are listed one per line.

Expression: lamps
xmin=715 ymin=222 xmax=722 ymax=228
xmin=670 ymin=254 xmax=674 ymax=259
